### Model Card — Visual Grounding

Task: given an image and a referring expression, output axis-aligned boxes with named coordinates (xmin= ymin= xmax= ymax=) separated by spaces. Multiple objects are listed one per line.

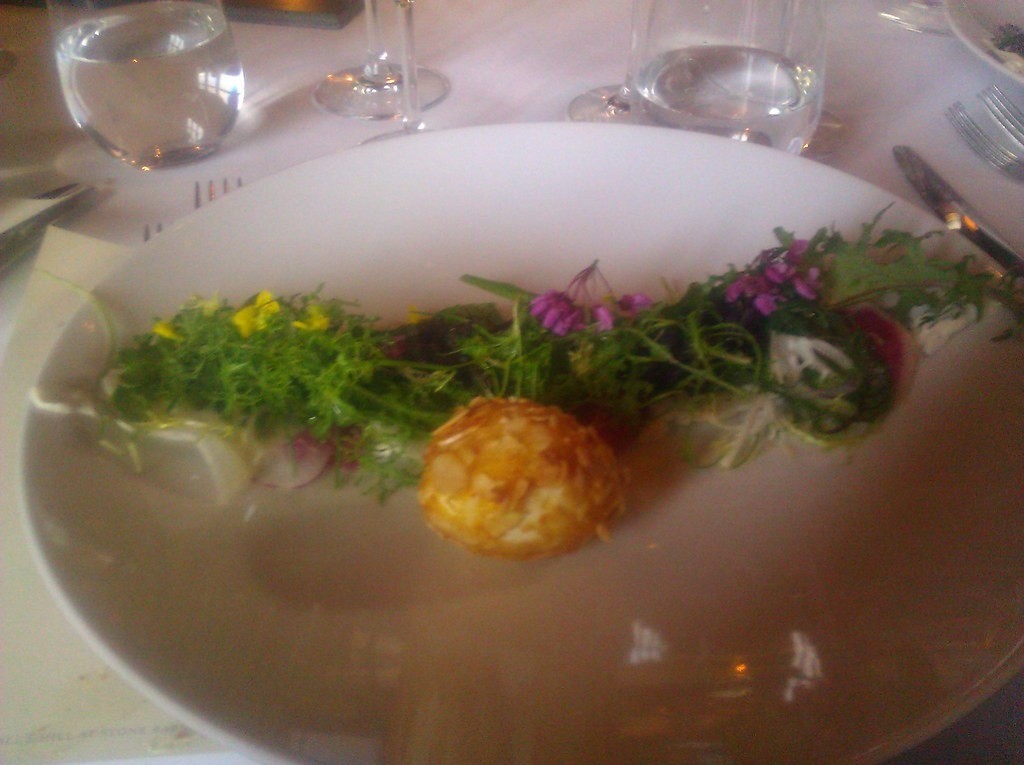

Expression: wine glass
xmin=566 ymin=0 xmax=659 ymax=127
xmin=358 ymin=0 xmax=435 ymax=146
xmin=311 ymin=0 xmax=451 ymax=120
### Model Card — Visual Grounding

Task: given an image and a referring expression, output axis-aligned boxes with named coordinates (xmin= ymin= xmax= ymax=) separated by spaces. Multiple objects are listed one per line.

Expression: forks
xmin=978 ymin=83 xmax=1024 ymax=150
xmin=944 ymin=100 xmax=1024 ymax=181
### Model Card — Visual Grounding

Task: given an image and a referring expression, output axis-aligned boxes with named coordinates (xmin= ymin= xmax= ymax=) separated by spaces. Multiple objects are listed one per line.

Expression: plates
xmin=940 ymin=0 xmax=1024 ymax=84
xmin=21 ymin=118 xmax=1024 ymax=765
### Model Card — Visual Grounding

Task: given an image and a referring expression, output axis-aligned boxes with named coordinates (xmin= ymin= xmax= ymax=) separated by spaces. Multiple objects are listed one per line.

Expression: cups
xmin=632 ymin=0 xmax=830 ymax=157
xmin=44 ymin=0 xmax=246 ymax=172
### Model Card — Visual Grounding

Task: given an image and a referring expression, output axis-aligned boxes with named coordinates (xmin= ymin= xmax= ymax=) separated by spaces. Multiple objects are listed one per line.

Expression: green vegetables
xmin=27 ymin=197 xmax=1024 ymax=505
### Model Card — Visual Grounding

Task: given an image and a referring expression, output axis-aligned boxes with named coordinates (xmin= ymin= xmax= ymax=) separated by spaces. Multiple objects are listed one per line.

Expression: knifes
xmin=893 ymin=145 xmax=1024 ymax=278
